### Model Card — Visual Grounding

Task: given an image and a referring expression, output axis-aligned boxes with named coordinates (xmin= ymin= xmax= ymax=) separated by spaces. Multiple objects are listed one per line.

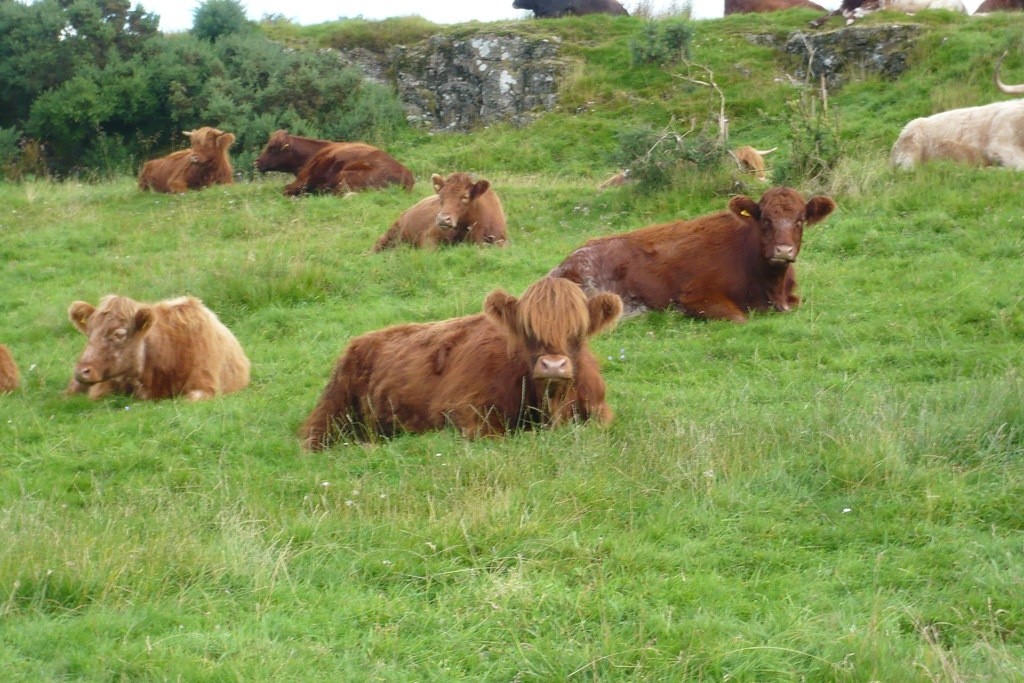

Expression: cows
xmin=140 ymin=126 xmax=236 ymax=194
xmin=301 ymin=277 xmax=624 ymax=453
xmin=545 ymin=187 xmax=836 ymax=323
xmin=65 ymin=293 xmax=251 ymax=400
xmin=253 ymin=131 xmax=414 ymax=197
xmin=375 ymin=172 xmax=507 ymax=252
xmin=0 ymin=341 xmax=23 ymax=390
xmin=889 ymin=50 xmax=1023 ymax=170
xmin=511 ymin=0 xmax=1023 ymax=18
